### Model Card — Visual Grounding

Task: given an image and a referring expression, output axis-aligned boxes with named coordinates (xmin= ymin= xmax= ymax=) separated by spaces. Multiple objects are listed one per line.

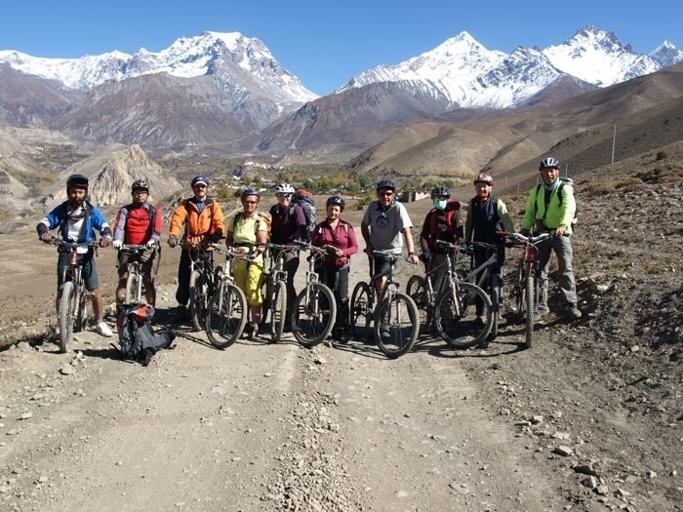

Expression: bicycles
xmin=497 ymin=227 xmax=570 ymax=349
xmin=291 ymin=240 xmax=336 ymax=349
xmin=39 ymin=234 xmax=101 ymax=354
xmin=122 ymin=242 xmax=148 ymax=317
xmin=173 ymin=236 xmax=227 ymax=332
xmin=350 ymin=247 xmax=420 ymax=359
xmin=407 ymin=238 xmax=494 ymax=347
xmin=446 ymin=241 xmax=506 ymax=337
xmin=254 ymin=237 xmax=305 ymax=342
xmin=205 ymin=242 xmax=257 ymax=349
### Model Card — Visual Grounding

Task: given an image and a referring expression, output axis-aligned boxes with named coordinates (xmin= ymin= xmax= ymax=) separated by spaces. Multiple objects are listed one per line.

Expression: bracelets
xmin=408 ymin=252 xmax=417 ymax=257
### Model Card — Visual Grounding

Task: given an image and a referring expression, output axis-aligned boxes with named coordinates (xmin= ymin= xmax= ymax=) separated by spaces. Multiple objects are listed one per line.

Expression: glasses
xmin=379 ymin=192 xmax=393 ymax=196
xmin=244 ymin=201 xmax=257 ymax=204
xmin=278 ymin=194 xmax=291 ymax=198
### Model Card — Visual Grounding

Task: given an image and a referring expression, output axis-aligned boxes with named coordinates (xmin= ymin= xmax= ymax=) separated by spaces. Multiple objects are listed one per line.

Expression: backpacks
xmin=274 ymin=189 xmax=316 ymax=244
xmin=232 ymin=212 xmax=272 ymax=258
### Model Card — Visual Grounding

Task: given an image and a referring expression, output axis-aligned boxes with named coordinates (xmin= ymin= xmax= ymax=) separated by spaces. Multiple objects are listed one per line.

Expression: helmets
xmin=376 ymin=180 xmax=396 ymax=191
xmin=190 ymin=176 xmax=210 ymax=188
xmin=539 ymin=157 xmax=560 ymax=171
xmin=131 ymin=180 xmax=150 ymax=194
xmin=473 ymin=173 xmax=494 ymax=186
xmin=67 ymin=174 xmax=89 ymax=192
xmin=241 ymin=189 xmax=260 ymax=201
xmin=326 ymin=196 xmax=345 ymax=209
xmin=431 ymin=187 xmax=451 ymax=198
xmin=275 ymin=182 xmax=296 ymax=195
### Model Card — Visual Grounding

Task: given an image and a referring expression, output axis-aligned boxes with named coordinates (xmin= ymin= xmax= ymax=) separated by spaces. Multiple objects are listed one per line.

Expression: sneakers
xmin=380 ymin=322 xmax=391 ymax=339
xmin=534 ymin=306 xmax=550 ymax=320
xmin=96 ymin=321 xmax=113 ymax=337
xmin=567 ymin=305 xmax=582 ymax=319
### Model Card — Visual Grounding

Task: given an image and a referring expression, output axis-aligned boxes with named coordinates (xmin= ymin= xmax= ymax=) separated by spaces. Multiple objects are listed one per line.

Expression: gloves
xmin=168 ymin=236 xmax=178 ymax=247
xmin=100 ymin=238 xmax=110 ymax=247
xmin=146 ymin=238 xmax=158 ymax=248
xmin=112 ymin=240 xmax=123 ymax=250
xmin=40 ymin=232 xmax=53 ymax=242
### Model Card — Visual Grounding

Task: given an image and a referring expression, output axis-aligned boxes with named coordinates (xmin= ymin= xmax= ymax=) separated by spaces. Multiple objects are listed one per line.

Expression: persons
xmin=37 ymin=175 xmax=114 ymax=338
xmin=112 ymin=180 xmax=163 ymax=324
xmin=169 ymin=176 xmax=225 ymax=322
xmin=420 ymin=187 xmax=465 ymax=334
xmin=311 ymin=196 xmax=357 ymax=343
xmin=519 ymin=157 xmax=582 ymax=319
xmin=361 ymin=180 xmax=419 ymax=338
xmin=225 ymin=189 xmax=267 ymax=339
xmin=465 ymin=174 xmax=516 ymax=330
xmin=269 ymin=182 xmax=310 ymax=333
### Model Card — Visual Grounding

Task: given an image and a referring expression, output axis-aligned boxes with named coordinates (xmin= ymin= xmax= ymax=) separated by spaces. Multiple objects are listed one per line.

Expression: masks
xmin=434 ymin=199 xmax=447 ymax=210
